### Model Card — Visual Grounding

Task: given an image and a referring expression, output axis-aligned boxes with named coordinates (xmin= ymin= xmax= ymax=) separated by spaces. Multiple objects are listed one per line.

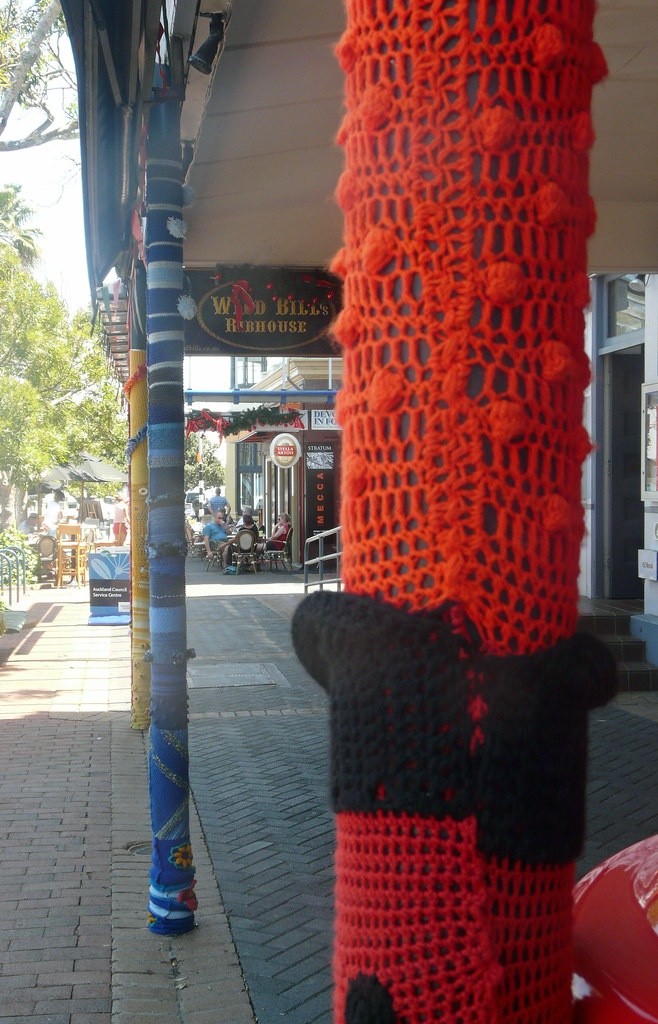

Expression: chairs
xmin=56 ymin=522 xmax=127 ymax=589
xmin=185 ymin=515 xmax=293 ymax=575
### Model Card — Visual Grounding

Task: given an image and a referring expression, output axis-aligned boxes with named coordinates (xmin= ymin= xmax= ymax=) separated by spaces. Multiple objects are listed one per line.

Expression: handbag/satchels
xmin=222 ymin=566 xmax=242 ymax=575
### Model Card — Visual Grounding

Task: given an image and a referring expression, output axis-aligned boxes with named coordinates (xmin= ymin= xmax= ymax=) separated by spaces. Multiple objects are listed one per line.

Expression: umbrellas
xmin=29 ymin=480 xmax=63 ymax=529
xmin=45 ymin=452 xmax=127 ymax=523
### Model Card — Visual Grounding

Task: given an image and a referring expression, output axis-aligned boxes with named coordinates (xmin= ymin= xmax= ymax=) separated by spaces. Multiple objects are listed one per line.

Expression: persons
xmin=208 ymin=488 xmax=231 ymax=523
xmin=192 ymin=496 xmax=199 ymax=518
xmin=203 ymin=498 xmax=210 ymax=514
xmin=18 ymin=513 xmax=47 ymax=533
xmin=43 ymin=490 xmax=64 ymax=538
xmin=203 ymin=510 xmax=232 ymax=570
xmin=218 ymin=507 xmax=258 ymax=574
xmin=114 ymin=494 xmax=130 ymax=540
xmin=255 ymin=513 xmax=290 ymax=570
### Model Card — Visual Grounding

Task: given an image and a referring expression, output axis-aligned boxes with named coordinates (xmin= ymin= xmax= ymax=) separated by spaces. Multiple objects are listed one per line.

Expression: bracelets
xmin=225 ymin=543 xmax=227 ymax=545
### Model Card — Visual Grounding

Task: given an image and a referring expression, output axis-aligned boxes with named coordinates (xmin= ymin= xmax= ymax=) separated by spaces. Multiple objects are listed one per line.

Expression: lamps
xmin=188 ymin=20 xmax=225 ymax=77
xmin=629 ymin=274 xmax=650 ymax=292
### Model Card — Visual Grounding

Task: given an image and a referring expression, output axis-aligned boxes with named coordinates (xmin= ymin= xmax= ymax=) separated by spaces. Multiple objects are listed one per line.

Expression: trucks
xmin=184 ymin=486 xmax=226 ymax=511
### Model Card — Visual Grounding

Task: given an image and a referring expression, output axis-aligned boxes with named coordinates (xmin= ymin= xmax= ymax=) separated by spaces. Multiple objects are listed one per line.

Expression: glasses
xmin=278 ymin=518 xmax=283 ymax=521
xmin=216 ymin=515 xmax=224 ymax=519
